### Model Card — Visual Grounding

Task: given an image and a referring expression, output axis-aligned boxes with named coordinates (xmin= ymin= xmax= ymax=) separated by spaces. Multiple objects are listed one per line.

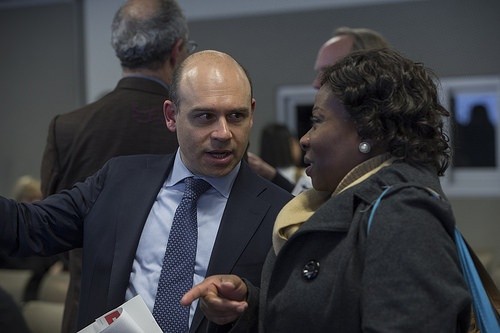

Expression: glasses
xmin=183 ymin=40 xmax=198 ymax=54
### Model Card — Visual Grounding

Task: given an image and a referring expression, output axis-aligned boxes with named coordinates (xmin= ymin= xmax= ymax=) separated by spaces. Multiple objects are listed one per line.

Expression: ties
xmin=152 ymin=176 xmax=213 ymax=333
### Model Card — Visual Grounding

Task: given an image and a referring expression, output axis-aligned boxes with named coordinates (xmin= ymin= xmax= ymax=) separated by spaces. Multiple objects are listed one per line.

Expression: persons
xmin=11 ymin=174 xmax=70 ymax=274
xmin=244 ymin=25 xmax=392 ymax=196
xmin=40 ymin=1 xmax=198 ymax=201
xmin=296 ymin=90 xmax=500 ymax=174
xmin=0 ymin=49 xmax=297 ymax=333
xmin=257 ymin=121 xmax=303 ymax=186
xmin=180 ymin=47 xmax=475 ymax=333
